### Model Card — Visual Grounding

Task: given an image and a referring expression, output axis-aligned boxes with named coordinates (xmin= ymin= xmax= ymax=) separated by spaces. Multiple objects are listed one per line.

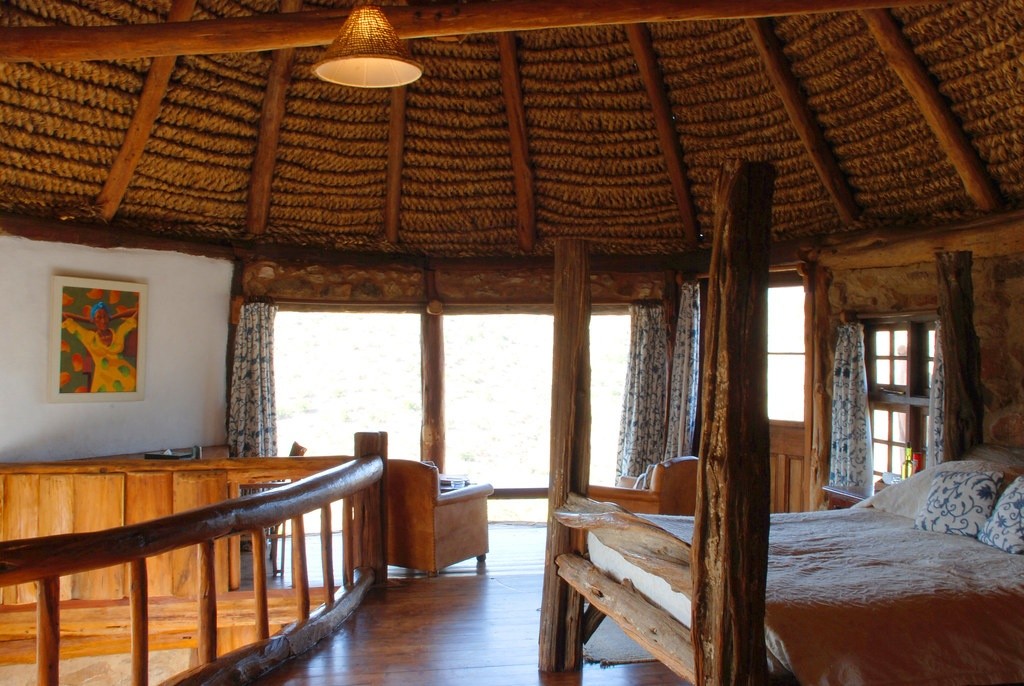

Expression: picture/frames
xmin=45 ymin=276 xmax=150 ymax=404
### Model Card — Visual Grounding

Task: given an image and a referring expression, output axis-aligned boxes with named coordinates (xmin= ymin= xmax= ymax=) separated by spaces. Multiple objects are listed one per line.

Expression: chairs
xmin=386 ymin=458 xmax=494 ymax=577
xmin=241 ymin=442 xmax=309 ymax=579
xmin=589 ymin=455 xmax=700 ymax=516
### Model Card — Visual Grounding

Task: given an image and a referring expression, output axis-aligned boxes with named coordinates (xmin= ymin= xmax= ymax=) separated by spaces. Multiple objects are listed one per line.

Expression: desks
xmin=820 ymin=483 xmax=890 ymax=513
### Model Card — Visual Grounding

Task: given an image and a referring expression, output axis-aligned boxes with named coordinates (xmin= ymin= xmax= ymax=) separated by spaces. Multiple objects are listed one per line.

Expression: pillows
xmin=634 ymin=465 xmax=656 ymax=490
xmin=978 ymin=474 xmax=1024 ymax=553
xmin=911 ymin=468 xmax=1005 ymax=539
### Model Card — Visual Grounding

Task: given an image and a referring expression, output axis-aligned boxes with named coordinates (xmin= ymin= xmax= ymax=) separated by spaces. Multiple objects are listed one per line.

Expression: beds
xmin=537 ymin=158 xmax=1024 ymax=686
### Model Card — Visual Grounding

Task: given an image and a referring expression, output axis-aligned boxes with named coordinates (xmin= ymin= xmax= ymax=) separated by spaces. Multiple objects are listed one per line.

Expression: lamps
xmin=308 ymin=6 xmax=425 ymax=89
